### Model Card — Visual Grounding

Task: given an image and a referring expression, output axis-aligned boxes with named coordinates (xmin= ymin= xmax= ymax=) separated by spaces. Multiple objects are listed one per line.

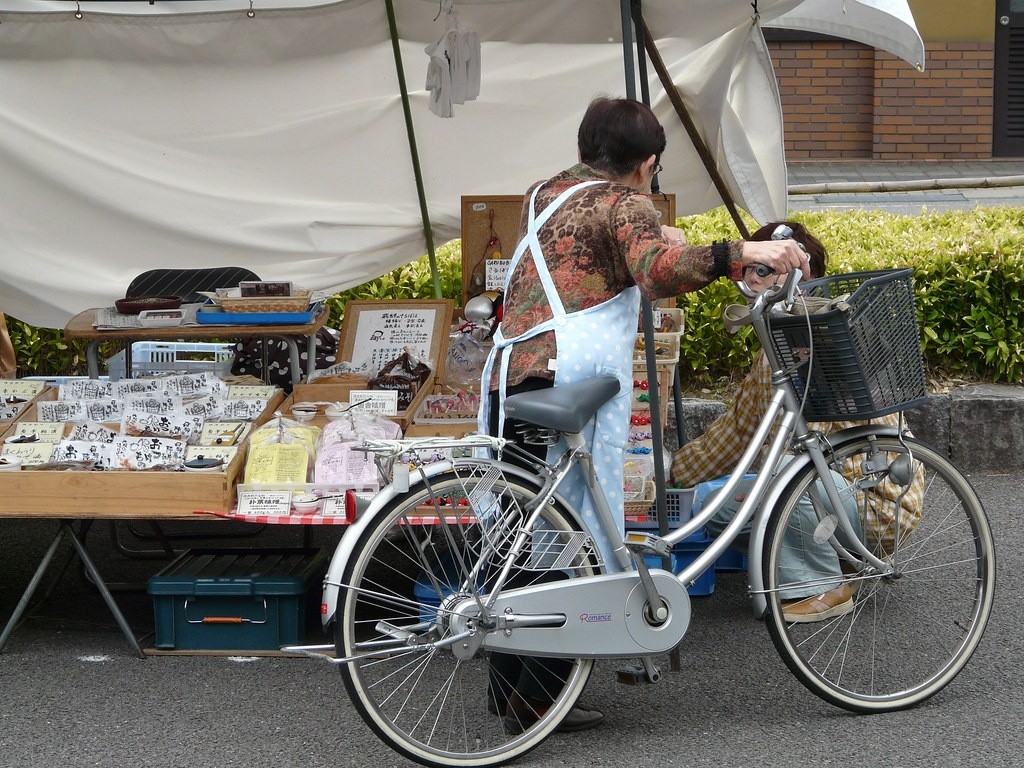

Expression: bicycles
xmin=281 ymin=239 xmax=999 ymax=768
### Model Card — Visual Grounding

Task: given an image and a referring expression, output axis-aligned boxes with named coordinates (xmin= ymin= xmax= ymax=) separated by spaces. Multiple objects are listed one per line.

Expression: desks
xmin=65 ymin=302 xmax=330 ymax=379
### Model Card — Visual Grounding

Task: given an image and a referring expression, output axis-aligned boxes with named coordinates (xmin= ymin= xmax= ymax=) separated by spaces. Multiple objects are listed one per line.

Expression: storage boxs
xmin=0 ymin=381 xmax=283 ymax=516
xmin=636 ymin=308 xmax=687 ymax=336
xmin=632 ymin=337 xmax=681 ymax=386
xmin=459 ymin=193 xmax=679 ymax=305
xmin=147 ymin=547 xmax=329 ymax=648
xmin=218 ymin=289 xmax=314 ymax=312
xmin=623 ymin=475 xmax=645 ymax=499
xmin=103 ymin=341 xmax=236 ymax=380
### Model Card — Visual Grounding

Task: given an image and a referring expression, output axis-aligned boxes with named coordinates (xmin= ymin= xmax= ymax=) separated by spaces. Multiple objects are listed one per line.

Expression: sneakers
xmin=779 ymin=578 xmax=855 ymax=623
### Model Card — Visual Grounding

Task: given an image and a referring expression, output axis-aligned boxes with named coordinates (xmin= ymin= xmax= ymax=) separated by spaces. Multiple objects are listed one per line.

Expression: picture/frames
xmin=337 ymin=298 xmax=453 ymax=385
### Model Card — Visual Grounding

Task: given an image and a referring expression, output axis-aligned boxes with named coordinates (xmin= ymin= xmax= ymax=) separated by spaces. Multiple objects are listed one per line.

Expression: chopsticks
xmin=309 ymin=495 xmax=344 ymax=502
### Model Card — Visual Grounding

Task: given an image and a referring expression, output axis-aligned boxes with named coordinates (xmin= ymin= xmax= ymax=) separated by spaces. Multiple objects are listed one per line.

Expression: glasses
xmin=744 ymin=262 xmax=757 ymax=276
xmin=649 ymin=162 xmax=664 ymax=177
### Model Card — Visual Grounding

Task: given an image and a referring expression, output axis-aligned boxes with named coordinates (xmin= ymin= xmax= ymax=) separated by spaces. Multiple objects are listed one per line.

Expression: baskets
xmin=623 ymin=481 xmax=657 ymax=516
xmin=633 ymin=308 xmax=684 ymax=387
xmin=624 ymin=484 xmax=698 ymax=528
xmin=218 ymin=289 xmax=315 ymax=312
xmin=765 ymin=265 xmax=927 ymax=422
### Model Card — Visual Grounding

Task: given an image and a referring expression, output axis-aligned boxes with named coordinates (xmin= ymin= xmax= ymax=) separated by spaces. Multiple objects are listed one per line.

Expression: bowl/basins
xmin=325 ymin=409 xmax=348 ymax=421
xmin=293 ymin=494 xmax=318 ymax=513
xmin=292 ymin=408 xmax=317 ymax=421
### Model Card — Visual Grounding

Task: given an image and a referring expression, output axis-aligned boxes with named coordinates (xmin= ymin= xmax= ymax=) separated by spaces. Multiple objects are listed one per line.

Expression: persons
xmin=670 ymin=220 xmax=924 ymax=622
xmin=487 ymin=92 xmax=810 ymax=735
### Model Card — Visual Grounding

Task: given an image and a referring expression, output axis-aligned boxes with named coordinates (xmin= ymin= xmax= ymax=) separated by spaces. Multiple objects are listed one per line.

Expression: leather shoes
xmin=488 ymin=683 xmax=513 ymax=716
xmin=504 ymin=692 xmax=605 ymax=734
